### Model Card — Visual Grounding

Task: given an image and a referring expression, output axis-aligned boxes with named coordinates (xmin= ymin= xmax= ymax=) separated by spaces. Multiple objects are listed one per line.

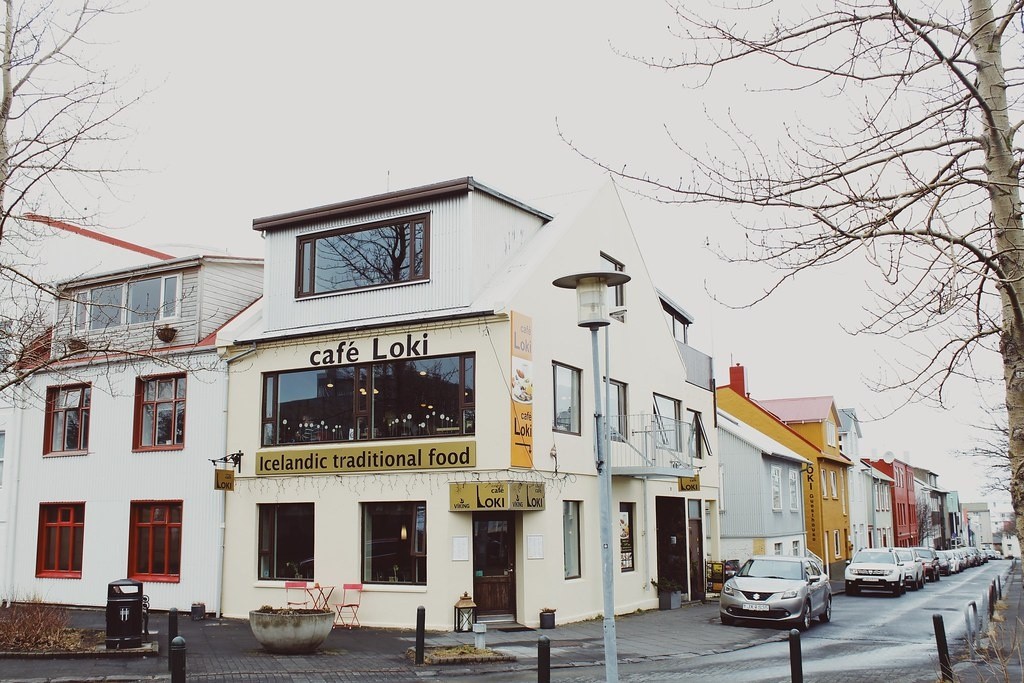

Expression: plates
xmin=512 ymin=395 xmax=532 ymax=404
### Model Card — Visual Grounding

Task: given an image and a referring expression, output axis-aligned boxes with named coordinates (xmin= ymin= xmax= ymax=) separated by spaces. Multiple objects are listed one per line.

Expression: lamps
xmin=453 ymin=591 xmax=477 ymax=633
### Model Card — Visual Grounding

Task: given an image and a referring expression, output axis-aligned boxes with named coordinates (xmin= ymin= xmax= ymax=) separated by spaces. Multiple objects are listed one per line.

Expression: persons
xmin=295 ymin=413 xmax=321 ymax=443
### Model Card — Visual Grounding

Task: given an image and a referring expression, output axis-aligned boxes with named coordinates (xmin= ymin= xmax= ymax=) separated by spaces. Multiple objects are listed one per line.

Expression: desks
xmin=304 ymin=586 xmax=336 ymax=629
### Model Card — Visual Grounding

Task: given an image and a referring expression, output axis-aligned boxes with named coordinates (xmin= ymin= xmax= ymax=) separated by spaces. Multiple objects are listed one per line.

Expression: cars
xmin=718 ymin=556 xmax=833 ymax=631
xmin=844 ymin=547 xmax=907 ymax=598
xmin=886 ymin=546 xmax=1002 ymax=591
xmin=299 ymin=537 xmax=411 ymax=582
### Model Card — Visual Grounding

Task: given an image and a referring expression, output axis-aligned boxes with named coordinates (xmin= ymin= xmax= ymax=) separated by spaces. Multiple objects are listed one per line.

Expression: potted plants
xmin=249 ymin=606 xmax=335 ymax=653
xmin=190 ymin=603 xmax=206 ymax=620
xmin=540 ymin=607 xmax=557 ymax=628
xmin=651 ymin=577 xmax=683 ymax=610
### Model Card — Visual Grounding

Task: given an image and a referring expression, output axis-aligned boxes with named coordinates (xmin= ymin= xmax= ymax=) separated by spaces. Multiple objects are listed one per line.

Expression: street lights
xmin=551 ymin=270 xmax=633 ymax=683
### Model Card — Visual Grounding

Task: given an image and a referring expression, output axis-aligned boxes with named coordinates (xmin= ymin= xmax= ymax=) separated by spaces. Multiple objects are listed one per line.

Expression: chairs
xmin=285 ymin=581 xmax=310 ymax=612
xmin=333 ymin=583 xmax=363 ymax=630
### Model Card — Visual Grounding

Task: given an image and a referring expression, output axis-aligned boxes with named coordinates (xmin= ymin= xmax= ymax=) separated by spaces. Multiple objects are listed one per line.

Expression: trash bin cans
xmin=104 ymin=578 xmax=144 ymax=649
